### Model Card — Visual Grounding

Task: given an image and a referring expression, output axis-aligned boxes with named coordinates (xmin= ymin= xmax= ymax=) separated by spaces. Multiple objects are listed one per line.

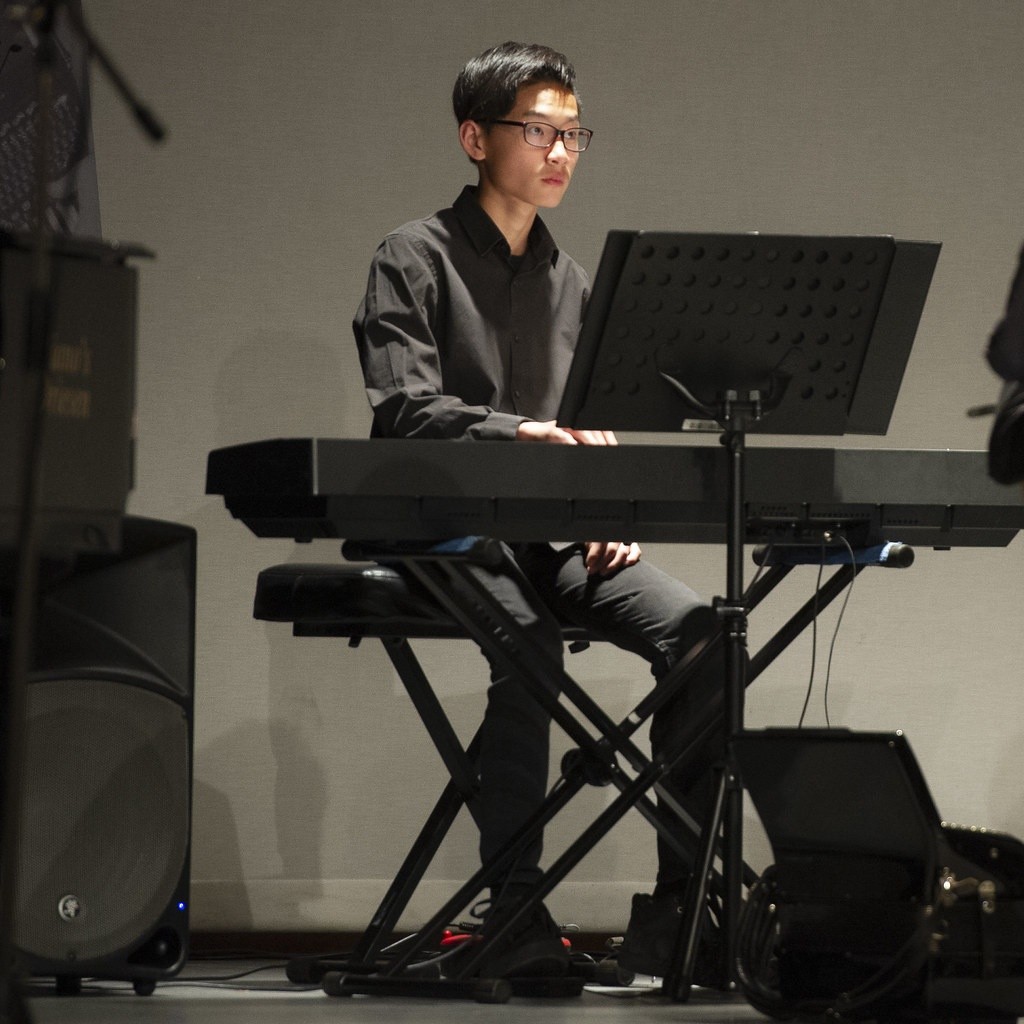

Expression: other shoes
xmin=617 ymin=893 xmax=743 ymax=993
xmin=484 ymin=902 xmax=569 ymax=977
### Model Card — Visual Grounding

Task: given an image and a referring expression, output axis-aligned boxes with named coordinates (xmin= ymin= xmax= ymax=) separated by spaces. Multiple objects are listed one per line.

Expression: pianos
xmin=203 ymin=430 xmax=1021 ymax=1001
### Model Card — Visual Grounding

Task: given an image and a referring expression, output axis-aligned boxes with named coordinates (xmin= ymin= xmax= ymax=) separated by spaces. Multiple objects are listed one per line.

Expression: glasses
xmin=475 ymin=118 xmax=594 ymax=153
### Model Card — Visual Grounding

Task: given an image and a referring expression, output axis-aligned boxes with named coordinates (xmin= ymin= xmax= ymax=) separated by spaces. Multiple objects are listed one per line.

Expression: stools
xmin=253 ymin=561 xmax=633 ymax=989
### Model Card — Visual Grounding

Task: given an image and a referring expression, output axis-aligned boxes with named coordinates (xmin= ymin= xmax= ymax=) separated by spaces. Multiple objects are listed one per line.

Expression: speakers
xmin=1 ymin=509 xmax=199 ymax=995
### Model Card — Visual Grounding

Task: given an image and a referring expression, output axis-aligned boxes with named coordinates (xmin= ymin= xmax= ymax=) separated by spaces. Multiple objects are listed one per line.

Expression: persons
xmin=350 ymin=41 xmax=748 ymax=996
